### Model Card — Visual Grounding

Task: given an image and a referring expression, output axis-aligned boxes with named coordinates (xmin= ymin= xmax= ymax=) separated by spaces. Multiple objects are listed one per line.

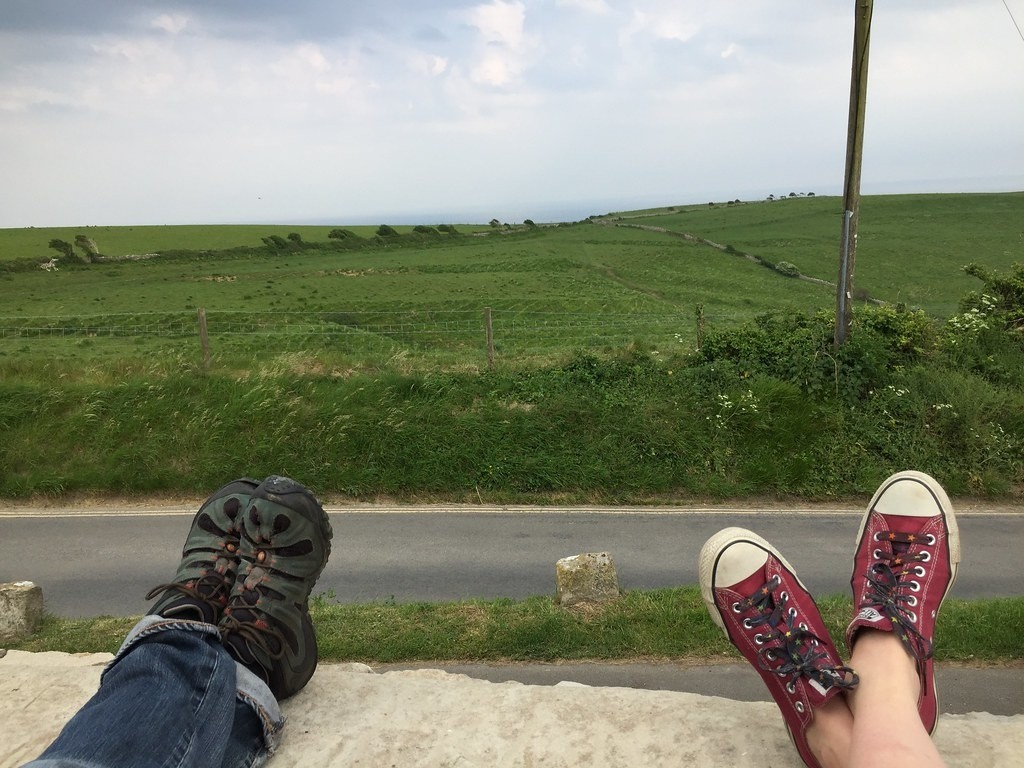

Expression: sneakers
xmin=699 ymin=526 xmax=859 ymax=768
xmin=146 ymin=477 xmax=262 ymax=625
xmin=845 ymin=470 xmax=960 ymax=739
xmin=217 ymin=476 xmax=334 ymax=703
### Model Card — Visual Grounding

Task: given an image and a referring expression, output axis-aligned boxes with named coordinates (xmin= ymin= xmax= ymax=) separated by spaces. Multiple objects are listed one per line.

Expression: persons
xmin=19 ymin=475 xmax=333 ymax=768
xmin=699 ymin=470 xmax=961 ymax=768
